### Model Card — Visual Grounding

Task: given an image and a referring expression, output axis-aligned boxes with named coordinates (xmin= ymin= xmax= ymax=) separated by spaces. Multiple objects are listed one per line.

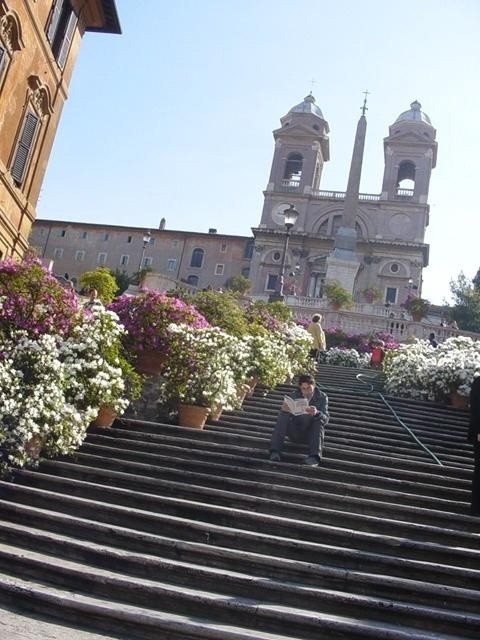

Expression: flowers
xmin=383 ymin=337 xmax=480 ymax=408
xmin=145 ymin=284 xmax=314 ymax=420
xmin=0 ymin=243 xmax=145 ymax=476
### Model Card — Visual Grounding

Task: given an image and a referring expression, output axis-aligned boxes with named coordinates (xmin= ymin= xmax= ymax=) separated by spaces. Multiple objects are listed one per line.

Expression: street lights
xmin=137 ymin=229 xmax=152 ymax=281
xmin=272 ymin=204 xmax=299 ymax=296
xmin=408 ymin=276 xmax=413 ymax=296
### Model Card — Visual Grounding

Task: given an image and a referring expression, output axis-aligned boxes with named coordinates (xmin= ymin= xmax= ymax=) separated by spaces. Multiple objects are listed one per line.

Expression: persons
xmin=269 ymin=373 xmax=330 ymax=466
xmin=428 ymin=332 xmax=439 ymax=348
xmin=306 ymin=311 xmax=327 ymax=364
xmin=466 ymin=375 xmax=480 ymax=517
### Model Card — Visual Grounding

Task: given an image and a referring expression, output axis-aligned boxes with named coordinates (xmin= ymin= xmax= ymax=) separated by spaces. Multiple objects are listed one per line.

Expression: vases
xmin=178 ymin=403 xmax=211 ymax=429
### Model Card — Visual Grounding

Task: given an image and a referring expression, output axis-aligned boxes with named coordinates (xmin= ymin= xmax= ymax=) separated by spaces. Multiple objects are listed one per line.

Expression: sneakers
xmin=270 ymin=451 xmax=280 ymax=461
xmin=304 ymin=459 xmax=319 ymax=467
